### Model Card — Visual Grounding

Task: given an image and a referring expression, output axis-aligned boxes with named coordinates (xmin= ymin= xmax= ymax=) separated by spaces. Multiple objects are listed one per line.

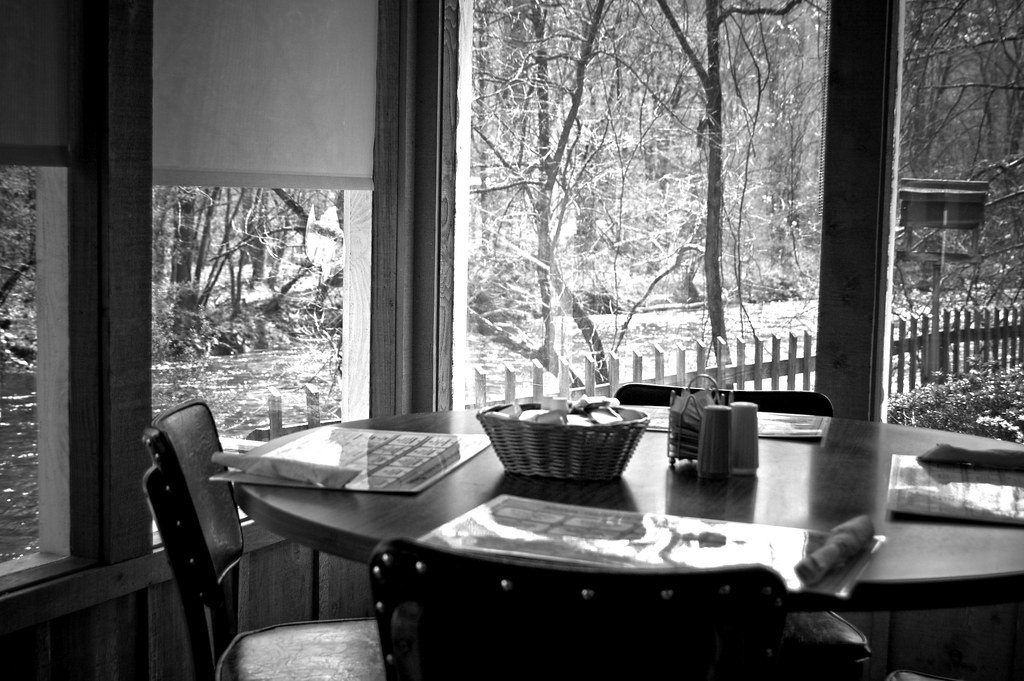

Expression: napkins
xmin=211 ymin=450 xmax=362 ymax=490
xmin=919 ymin=443 xmax=1024 ymax=469
xmin=794 ymin=510 xmax=875 ymax=586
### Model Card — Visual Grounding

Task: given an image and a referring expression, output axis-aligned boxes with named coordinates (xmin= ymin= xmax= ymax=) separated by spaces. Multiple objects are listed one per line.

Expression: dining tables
xmin=230 ymin=404 xmax=1024 ymax=681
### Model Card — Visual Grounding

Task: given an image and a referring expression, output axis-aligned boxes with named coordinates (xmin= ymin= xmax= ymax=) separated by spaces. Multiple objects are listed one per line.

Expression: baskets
xmin=478 ymin=402 xmax=650 ymax=480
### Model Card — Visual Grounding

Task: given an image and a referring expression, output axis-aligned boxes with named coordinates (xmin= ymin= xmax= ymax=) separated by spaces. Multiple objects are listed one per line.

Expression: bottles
xmin=696 ymin=405 xmax=733 ymax=480
xmin=728 ymin=401 xmax=760 ymax=476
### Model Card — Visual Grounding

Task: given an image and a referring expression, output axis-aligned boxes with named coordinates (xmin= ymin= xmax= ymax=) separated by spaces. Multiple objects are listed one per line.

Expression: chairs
xmin=366 ymin=533 xmax=787 ymax=680
xmin=613 ymin=384 xmax=873 ymax=680
xmin=140 ymin=401 xmax=388 ymax=681
